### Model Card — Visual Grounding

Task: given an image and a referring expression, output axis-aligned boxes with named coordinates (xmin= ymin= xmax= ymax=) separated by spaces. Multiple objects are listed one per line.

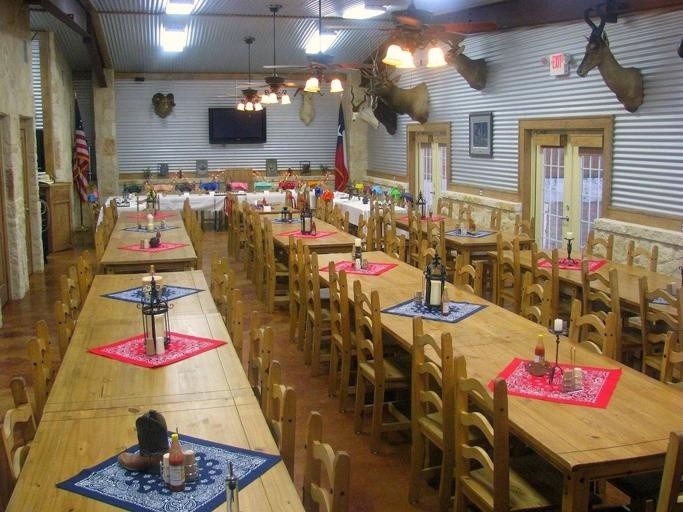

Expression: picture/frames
xmin=469 ymin=111 xmax=492 ymax=158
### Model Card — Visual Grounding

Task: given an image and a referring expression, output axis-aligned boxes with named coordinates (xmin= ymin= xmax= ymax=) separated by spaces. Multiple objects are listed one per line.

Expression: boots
xmin=118 ymin=410 xmax=168 ymax=472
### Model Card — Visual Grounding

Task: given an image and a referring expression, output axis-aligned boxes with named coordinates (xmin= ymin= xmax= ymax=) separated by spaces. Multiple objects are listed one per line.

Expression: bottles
xmin=149 ymin=265 xmax=155 ymax=277
xmin=183 ymin=448 xmax=197 ymax=483
xmin=441 ymin=286 xmax=449 ymax=316
xmin=415 ymin=292 xmax=421 ymax=308
xmin=454 ymin=223 xmax=477 ymax=236
xmin=562 ymin=367 xmax=583 ymax=391
xmin=146 ymin=338 xmax=153 ymax=355
xmin=261 ymin=196 xmax=272 ymax=212
xmin=168 ymin=434 xmax=187 ymax=493
xmin=136 ymin=213 xmax=165 ymax=249
xmin=351 ymin=244 xmax=368 ymax=270
xmin=535 ymin=334 xmax=545 ymax=362
xmin=162 ymin=453 xmax=170 ymax=485
xmin=310 ymin=222 xmax=315 ymax=235
xmin=155 ymin=336 xmax=164 ymax=355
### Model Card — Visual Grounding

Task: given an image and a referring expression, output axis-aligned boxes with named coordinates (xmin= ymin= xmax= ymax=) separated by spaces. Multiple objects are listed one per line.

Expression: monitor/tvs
xmin=208 ymin=107 xmax=266 ymax=145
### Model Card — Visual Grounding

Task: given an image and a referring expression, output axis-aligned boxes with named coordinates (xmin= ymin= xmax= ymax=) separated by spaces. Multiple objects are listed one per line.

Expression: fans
xmin=236 ymin=4 xmax=302 ymax=98
xmin=320 ymin=0 xmax=497 ymax=64
xmin=217 ymin=36 xmax=269 ymax=103
xmin=263 ymin=51 xmax=375 ymax=79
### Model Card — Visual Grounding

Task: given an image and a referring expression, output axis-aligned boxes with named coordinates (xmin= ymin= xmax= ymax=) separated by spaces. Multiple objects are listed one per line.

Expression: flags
xmin=334 ymin=94 xmax=350 ymax=191
xmin=73 ymin=91 xmax=90 ymax=202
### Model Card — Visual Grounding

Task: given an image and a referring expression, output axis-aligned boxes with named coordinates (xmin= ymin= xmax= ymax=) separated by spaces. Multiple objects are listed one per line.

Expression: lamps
xmin=261 ymin=93 xmax=291 ymax=104
xmin=304 ymin=77 xmax=344 ymax=93
xmin=382 ymin=45 xmax=447 ymax=68
xmin=237 ymin=102 xmax=263 ymax=112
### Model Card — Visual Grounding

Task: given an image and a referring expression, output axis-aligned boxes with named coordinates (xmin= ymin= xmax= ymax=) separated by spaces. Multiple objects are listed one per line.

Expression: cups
xmin=666 ymin=281 xmax=677 ymax=296
xmin=208 ymin=191 xmax=214 ymax=198
xmin=183 ymin=192 xmax=189 ymax=199
